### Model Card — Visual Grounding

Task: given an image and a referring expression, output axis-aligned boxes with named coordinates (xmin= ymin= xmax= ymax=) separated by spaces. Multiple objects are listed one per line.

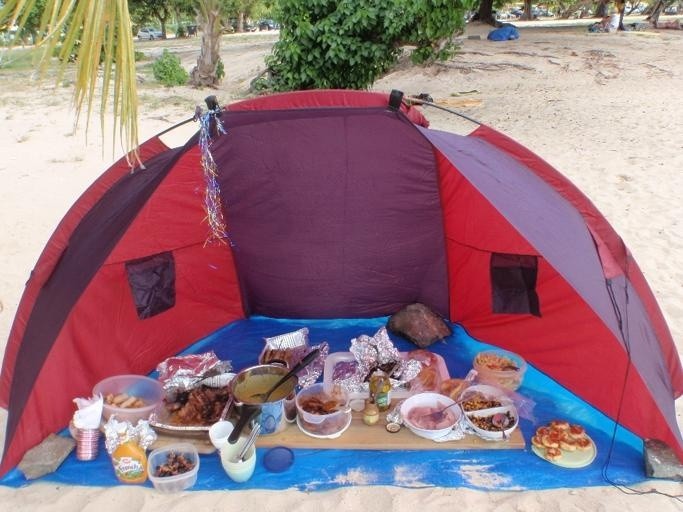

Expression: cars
xmin=230 ymin=15 xmax=283 ymax=34
xmin=136 ymin=26 xmax=165 ymax=41
xmin=462 ymin=2 xmax=681 ymax=22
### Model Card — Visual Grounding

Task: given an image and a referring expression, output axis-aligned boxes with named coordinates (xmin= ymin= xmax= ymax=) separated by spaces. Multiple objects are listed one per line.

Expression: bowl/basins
xmin=58 ymin=341 xmax=597 ymax=494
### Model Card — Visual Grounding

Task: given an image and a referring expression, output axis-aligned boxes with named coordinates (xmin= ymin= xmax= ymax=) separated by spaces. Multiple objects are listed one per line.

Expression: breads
xmin=531 ymin=421 xmax=592 ymax=461
xmin=104 ymin=392 xmax=144 ymax=408
xmin=409 ymin=350 xmax=470 ymax=402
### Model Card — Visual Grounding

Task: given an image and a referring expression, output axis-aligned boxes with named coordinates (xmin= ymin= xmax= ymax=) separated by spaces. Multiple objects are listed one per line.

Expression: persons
xmin=602 ymin=0 xmax=621 ymax=35
xmin=652 ymin=18 xmax=683 ymax=30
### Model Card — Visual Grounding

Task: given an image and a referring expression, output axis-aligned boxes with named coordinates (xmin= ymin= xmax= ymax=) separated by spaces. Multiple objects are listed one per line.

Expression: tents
xmin=0 ymin=87 xmax=683 ymax=494
